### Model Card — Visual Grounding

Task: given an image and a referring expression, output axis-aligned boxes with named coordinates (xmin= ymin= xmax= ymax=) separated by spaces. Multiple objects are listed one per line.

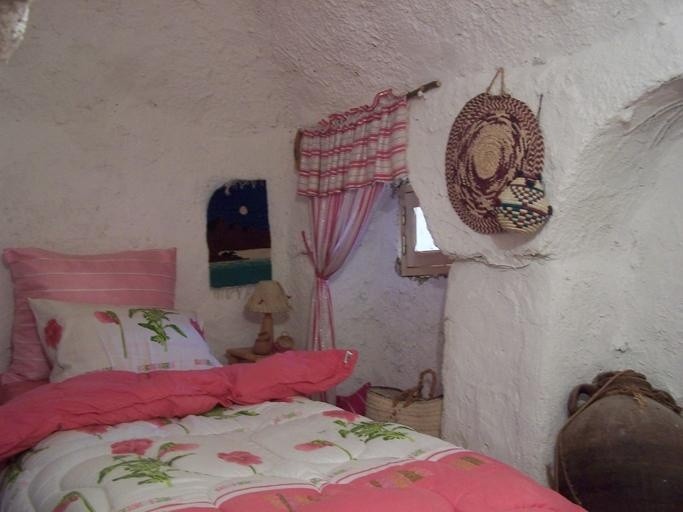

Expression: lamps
xmin=245 ymin=281 xmax=294 ymax=355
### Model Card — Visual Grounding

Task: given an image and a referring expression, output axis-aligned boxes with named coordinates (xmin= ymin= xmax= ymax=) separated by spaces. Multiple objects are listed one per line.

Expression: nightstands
xmin=227 ymin=346 xmax=282 ymax=362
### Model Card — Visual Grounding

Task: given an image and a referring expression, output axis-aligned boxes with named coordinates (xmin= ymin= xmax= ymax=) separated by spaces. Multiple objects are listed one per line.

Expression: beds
xmin=1 ymin=363 xmax=591 ymax=512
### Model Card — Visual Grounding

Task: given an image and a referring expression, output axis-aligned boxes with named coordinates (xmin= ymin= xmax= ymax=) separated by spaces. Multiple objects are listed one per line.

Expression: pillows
xmin=28 ymin=297 xmax=224 ymax=383
xmin=1 ymin=247 xmax=177 ymax=385
